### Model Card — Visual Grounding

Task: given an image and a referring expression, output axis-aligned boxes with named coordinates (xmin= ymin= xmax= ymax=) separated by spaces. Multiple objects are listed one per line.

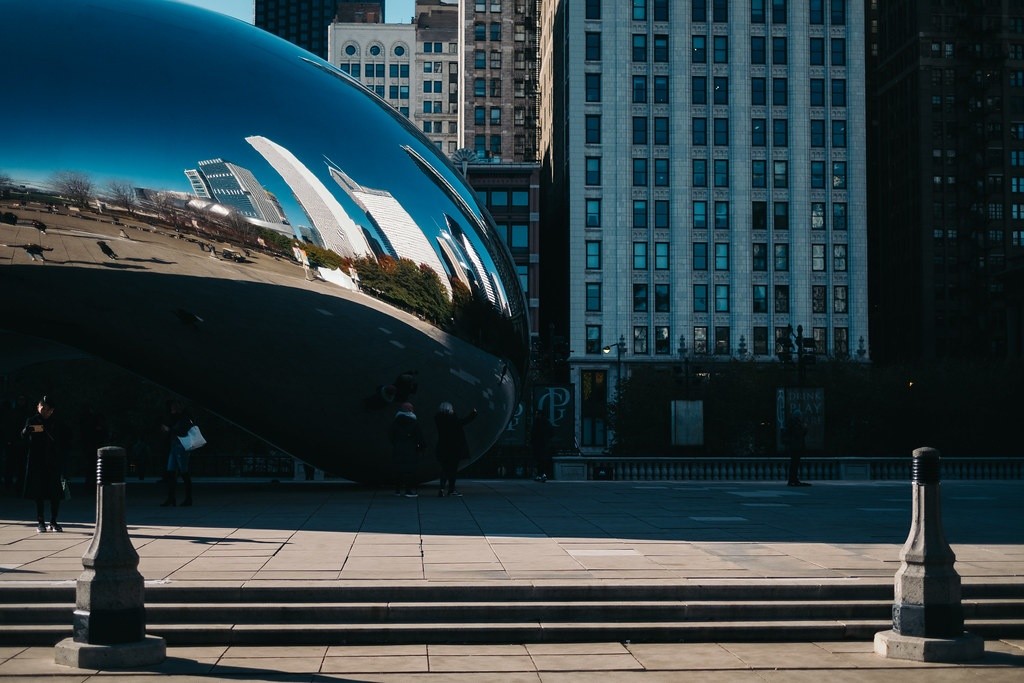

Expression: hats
xmin=439 ymin=402 xmax=453 ymax=416
xmin=793 ymin=410 xmax=800 ymax=417
xmin=401 ymin=403 xmax=412 ymax=412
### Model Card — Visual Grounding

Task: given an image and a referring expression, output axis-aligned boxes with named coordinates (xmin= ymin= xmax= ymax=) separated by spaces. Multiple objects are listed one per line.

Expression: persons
xmin=391 ymin=402 xmax=428 ymax=497
xmin=433 ymin=401 xmax=479 ymax=497
xmin=788 ymin=409 xmax=808 ymax=486
xmin=20 ymin=396 xmax=70 ymax=531
xmin=500 ymin=361 xmax=507 ymax=383
xmin=160 ymin=396 xmax=195 ymax=507
xmin=365 ymin=367 xmax=420 ymax=409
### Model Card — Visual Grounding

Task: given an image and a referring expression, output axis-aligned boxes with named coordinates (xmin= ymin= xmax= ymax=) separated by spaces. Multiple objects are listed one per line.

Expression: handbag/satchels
xmin=416 ymin=441 xmax=425 ymax=452
xmin=176 ymin=419 xmax=207 ymax=451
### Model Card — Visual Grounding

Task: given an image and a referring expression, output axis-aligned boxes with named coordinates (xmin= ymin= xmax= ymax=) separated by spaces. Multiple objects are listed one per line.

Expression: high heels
xmin=38 ymin=522 xmax=46 ymax=531
xmin=180 ymin=473 xmax=193 ymax=507
xmin=160 ymin=471 xmax=176 ymax=507
xmin=50 ymin=520 xmax=62 ymax=531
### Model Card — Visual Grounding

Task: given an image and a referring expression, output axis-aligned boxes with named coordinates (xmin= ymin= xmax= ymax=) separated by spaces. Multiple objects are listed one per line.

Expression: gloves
xmin=474 ymin=408 xmax=480 ymax=415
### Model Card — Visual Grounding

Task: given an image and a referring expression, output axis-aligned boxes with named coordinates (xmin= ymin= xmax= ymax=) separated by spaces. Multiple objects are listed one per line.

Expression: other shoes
xmin=788 ymin=481 xmax=802 ymax=487
xmin=395 ymin=490 xmax=400 ymax=496
xmin=534 ymin=474 xmax=547 ymax=482
xmin=447 ymin=490 xmax=464 ymax=496
xmin=404 ymin=489 xmax=418 ymax=497
xmin=439 ymin=488 xmax=444 ymax=497
